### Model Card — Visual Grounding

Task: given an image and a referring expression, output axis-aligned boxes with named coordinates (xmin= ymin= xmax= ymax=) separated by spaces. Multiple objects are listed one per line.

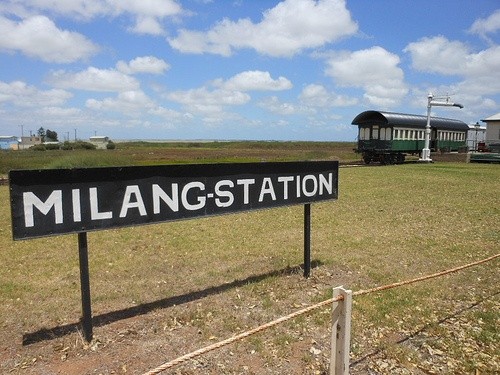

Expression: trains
xmin=351 ymin=111 xmax=469 ymax=163
